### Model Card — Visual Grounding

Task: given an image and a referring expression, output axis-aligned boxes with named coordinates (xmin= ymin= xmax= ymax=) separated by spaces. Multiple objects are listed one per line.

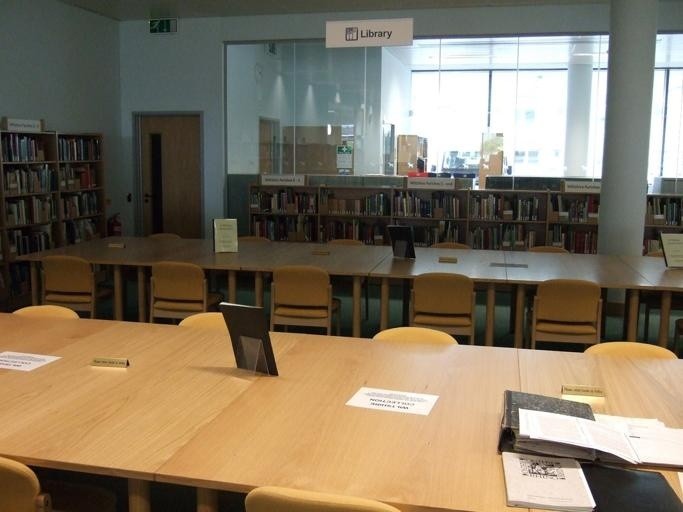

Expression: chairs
xmin=15 ymin=304 xmax=80 ymax=318
xmin=267 ymin=261 xmax=332 ymax=336
xmin=146 ymin=262 xmax=209 ymax=321
xmin=37 ymin=254 xmax=98 ymax=322
xmin=176 ymin=312 xmax=229 ymax=333
xmin=245 ymin=487 xmax=401 ymax=512
xmin=374 ymin=327 xmax=459 ymax=344
xmin=530 ymin=277 xmax=602 ymax=351
xmin=410 ymin=267 xmax=477 ymax=344
xmin=579 ymin=340 xmax=678 ymax=357
xmin=2 ymin=457 xmax=51 ymax=512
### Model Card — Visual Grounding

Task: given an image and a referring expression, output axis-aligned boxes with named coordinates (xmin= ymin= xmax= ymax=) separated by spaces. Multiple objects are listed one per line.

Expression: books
xmin=1 ymin=135 xmax=103 ymax=300
xmin=550 ymin=194 xmax=599 ymax=253
xmin=250 ymin=190 xmax=318 ymax=242
xmin=497 ymin=389 xmax=682 ymax=512
xmin=470 ymin=193 xmax=545 ymax=251
xmin=320 ymin=190 xmax=459 ymax=247
xmin=643 ymin=197 xmax=681 ymax=251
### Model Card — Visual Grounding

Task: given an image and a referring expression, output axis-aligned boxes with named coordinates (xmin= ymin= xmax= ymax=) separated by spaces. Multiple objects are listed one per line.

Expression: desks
xmin=1 ymin=314 xmax=682 ymax=512
xmin=10 ymin=235 xmax=682 ymax=359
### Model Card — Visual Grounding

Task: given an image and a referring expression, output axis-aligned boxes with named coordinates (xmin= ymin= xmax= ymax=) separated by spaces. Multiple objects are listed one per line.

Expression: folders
xmin=498 ymin=390 xmax=596 ymax=465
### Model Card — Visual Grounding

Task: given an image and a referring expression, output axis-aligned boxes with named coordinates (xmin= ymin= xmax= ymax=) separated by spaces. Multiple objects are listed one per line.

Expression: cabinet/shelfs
xmin=1 ymin=130 xmax=108 ymax=312
xmin=239 ymin=182 xmax=683 ymax=253
